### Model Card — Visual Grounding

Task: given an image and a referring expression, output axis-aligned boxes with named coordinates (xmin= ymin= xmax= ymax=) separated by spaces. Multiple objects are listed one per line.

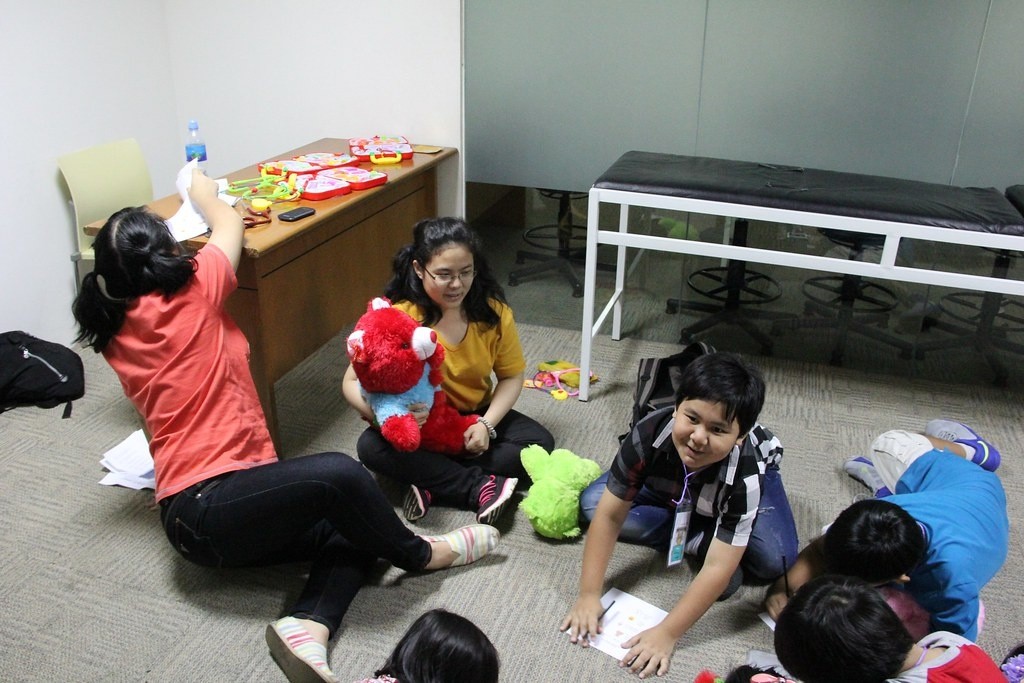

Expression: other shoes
xmin=697 ymin=531 xmax=743 ymax=601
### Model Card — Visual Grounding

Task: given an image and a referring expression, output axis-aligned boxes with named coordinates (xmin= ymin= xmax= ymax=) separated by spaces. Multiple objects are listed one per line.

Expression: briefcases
xmin=348 ymin=136 xmax=414 ymax=165
xmin=258 ymin=152 xmax=360 ymax=182
xmin=273 ymin=166 xmax=388 ymax=201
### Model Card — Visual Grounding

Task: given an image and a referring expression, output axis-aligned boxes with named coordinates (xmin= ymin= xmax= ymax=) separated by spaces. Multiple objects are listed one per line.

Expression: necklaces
xmin=915 ymin=647 xmax=927 ymax=667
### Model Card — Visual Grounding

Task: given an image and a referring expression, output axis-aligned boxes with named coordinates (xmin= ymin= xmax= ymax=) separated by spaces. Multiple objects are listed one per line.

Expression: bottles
xmin=185 ymin=120 xmax=207 ymax=176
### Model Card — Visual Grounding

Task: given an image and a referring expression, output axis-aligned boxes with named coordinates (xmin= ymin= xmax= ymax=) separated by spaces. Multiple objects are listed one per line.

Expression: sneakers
xmin=476 ymin=475 xmax=518 ymax=526
xmin=403 ymin=484 xmax=431 ymax=520
xmin=925 ymin=419 xmax=1001 ymax=472
xmin=415 ymin=525 xmax=500 ymax=567
xmin=843 ymin=455 xmax=893 ymax=498
xmin=266 ymin=616 xmax=340 ymax=683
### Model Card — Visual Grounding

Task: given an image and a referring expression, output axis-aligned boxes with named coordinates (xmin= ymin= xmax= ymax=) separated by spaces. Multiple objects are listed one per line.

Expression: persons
xmin=559 ymin=353 xmax=800 ymax=679
xmin=340 ymin=215 xmax=556 ymax=525
xmin=764 ymin=419 xmax=1009 ymax=682
xmin=72 ymin=164 xmax=501 ymax=683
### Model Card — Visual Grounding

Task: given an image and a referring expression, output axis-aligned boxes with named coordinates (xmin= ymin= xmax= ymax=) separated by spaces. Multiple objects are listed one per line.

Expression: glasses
xmin=423 ymin=266 xmax=477 ymax=285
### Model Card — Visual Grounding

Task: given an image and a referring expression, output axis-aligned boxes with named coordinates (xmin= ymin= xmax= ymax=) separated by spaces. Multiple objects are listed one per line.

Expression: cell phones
xmin=278 ymin=207 xmax=315 ymax=222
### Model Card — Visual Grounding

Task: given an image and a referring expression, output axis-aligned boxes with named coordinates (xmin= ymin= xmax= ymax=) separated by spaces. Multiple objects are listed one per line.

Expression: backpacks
xmin=0 ymin=331 xmax=84 ymax=419
xmin=618 ymin=342 xmax=717 ymax=446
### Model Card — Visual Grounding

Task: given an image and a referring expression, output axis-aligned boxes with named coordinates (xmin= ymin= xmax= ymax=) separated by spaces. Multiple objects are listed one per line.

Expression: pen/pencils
xmin=781 ymin=555 xmax=789 ymax=596
xmin=583 ymin=601 xmax=616 ymax=639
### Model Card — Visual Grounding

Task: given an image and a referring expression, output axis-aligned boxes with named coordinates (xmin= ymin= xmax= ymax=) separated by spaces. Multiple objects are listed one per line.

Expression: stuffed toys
xmin=727 ymin=665 xmax=796 ymax=683
xmin=519 ymin=445 xmax=604 ymax=540
xmin=347 ymin=295 xmax=483 ymax=457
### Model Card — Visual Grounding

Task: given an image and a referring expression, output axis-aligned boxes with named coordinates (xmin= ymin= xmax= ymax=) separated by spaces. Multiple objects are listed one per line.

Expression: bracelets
xmin=476 ymin=417 xmax=497 ymax=440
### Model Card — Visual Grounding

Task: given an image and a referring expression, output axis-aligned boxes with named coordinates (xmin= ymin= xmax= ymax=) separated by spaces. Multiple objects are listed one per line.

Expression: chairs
xmin=54 ymin=138 xmax=154 ymax=291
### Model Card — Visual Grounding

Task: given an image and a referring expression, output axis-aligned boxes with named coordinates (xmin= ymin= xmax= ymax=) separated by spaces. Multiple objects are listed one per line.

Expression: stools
xmin=508 ymin=183 xmax=1024 ymax=360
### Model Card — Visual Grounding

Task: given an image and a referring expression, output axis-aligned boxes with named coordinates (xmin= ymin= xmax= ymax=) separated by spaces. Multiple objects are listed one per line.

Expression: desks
xmin=82 ymin=140 xmax=457 ymax=460
xmin=574 ymin=150 xmax=1024 ymax=407
